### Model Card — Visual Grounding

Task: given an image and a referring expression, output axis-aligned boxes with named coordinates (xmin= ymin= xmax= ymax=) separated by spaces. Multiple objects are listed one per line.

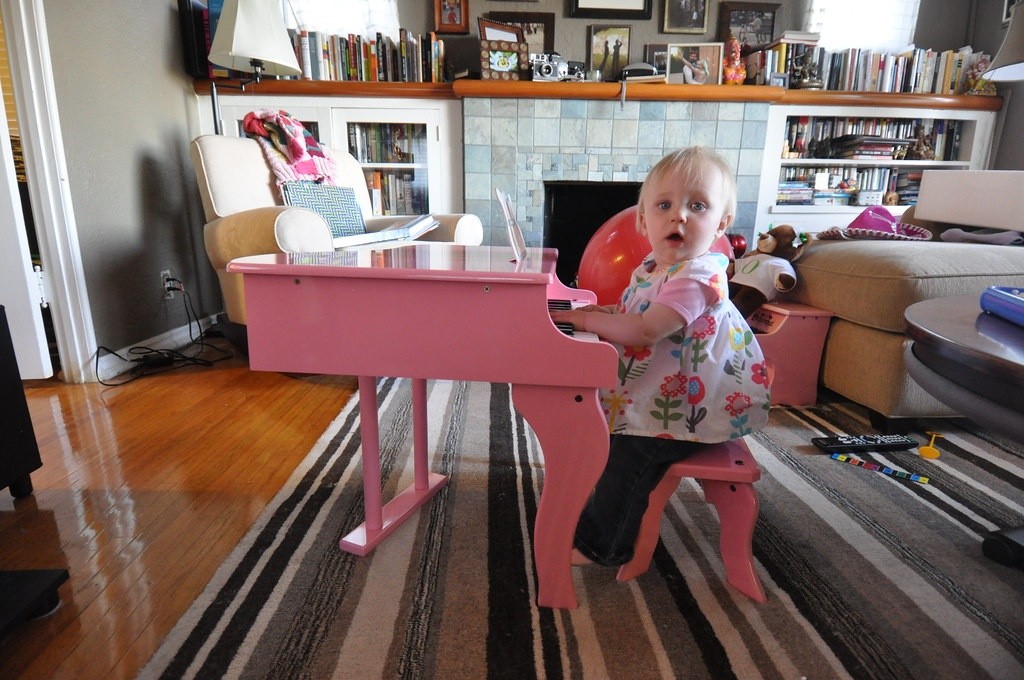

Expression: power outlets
xmin=160 ymin=270 xmax=175 ymax=300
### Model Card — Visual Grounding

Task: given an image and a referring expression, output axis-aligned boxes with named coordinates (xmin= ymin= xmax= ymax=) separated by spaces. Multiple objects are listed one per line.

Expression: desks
xmin=905 ymin=294 xmax=1024 ymax=567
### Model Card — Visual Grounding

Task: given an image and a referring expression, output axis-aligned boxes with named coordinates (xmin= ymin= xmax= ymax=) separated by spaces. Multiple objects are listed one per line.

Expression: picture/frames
xmin=480 ymin=38 xmax=528 ymax=80
xmin=588 ymin=23 xmax=633 ymax=81
xmin=717 ymin=2 xmax=782 ymax=50
xmin=666 ymin=43 xmax=724 ymax=86
xmin=487 ymin=10 xmax=556 ymax=57
xmin=478 ymin=15 xmax=524 ymax=47
xmin=434 ymin=0 xmax=471 ymax=33
xmin=645 ymin=44 xmax=666 ymax=81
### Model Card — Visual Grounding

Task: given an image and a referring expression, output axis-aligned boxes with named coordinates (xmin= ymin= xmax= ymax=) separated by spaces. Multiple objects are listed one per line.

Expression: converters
xmin=144 ymin=354 xmax=174 ymax=368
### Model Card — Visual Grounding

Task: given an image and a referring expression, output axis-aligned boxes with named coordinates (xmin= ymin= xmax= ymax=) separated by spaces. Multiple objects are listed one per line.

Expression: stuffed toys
xmin=726 ymin=222 xmax=813 ymax=318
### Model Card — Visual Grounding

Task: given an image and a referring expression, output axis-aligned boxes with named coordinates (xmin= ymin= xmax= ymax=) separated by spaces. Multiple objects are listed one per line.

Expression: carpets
xmin=131 ymin=374 xmax=1024 ymax=680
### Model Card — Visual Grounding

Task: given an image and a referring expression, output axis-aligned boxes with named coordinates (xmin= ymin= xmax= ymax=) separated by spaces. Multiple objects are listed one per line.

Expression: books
xmin=347 ymin=122 xmax=416 ymax=164
xmin=177 ymin=0 xmax=251 ymax=81
xmin=379 ymin=214 xmax=441 ymax=241
xmin=776 ymin=165 xmax=922 ymax=205
xmin=782 ymin=114 xmax=963 ymax=161
xmin=746 ymin=30 xmax=992 ymax=94
xmin=364 ymin=169 xmax=429 ymax=215
xmin=286 ymin=26 xmax=445 ymax=83
xmin=306 ymin=122 xmax=319 ymax=142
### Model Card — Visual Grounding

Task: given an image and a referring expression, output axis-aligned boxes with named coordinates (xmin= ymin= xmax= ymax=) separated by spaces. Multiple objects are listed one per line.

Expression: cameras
xmin=529 ymin=53 xmax=563 ymax=81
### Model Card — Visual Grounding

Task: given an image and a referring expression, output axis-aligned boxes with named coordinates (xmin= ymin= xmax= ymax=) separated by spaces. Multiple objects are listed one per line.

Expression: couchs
xmin=190 ymin=134 xmax=483 ymax=325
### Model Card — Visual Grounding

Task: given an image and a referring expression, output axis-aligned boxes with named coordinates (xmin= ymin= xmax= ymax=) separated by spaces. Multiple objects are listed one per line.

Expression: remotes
xmin=812 ymin=433 xmax=920 ymax=455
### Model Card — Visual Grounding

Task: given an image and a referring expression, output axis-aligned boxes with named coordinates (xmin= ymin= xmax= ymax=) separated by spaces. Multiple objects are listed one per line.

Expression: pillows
xmin=282 ymin=181 xmax=366 ymax=239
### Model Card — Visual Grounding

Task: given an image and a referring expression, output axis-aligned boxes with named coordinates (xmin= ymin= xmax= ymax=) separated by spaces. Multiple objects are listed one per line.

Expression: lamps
xmin=208 ymin=0 xmax=303 ymax=135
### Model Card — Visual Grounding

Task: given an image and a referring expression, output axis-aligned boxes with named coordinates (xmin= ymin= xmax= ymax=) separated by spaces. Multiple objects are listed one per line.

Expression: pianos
xmin=226 ymin=239 xmax=620 ymax=610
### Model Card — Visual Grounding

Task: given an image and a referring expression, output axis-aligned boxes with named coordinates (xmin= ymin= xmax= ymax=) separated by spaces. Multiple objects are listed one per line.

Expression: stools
xmin=783 ymin=204 xmax=1023 ymax=434
xmin=617 ymin=436 xmax=769 ymax=604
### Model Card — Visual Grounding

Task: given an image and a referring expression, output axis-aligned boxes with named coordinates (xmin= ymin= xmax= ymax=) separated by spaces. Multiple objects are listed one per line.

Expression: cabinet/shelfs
xmin=195 ymin=94 xmax=464 ymax=218
xmin=749 ymin=90 xmax=1002 ymax=247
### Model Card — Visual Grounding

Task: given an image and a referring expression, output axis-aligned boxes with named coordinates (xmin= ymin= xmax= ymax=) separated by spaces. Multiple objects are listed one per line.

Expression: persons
xmin=912 ymin=123 xmax=932 ymax=154
xmin=691 ymin=6 xmax=699 ymax=27
xmin=723 ymin=32 xmax=746 ymax=85
xmin=548 ymin=146 xmax=769 ymax=567
xmin=506 ymin=22 xmax=538 ymax=34
xmin=739 ymin=14 xmax=763 ymax=44
xmin=447 ymin=7 xmax=457 ymax=25
xmin=966 ymin=58 xmax=990 ymax=90
xmin=799 ymin=47 xmax=814 ymax=82
xmin=677 ymin=48 xmax=710 ymax=84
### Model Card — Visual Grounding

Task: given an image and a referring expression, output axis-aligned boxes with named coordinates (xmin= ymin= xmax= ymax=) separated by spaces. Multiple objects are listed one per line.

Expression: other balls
xmin=577 ymin=203 xmax=738 ymax=306
